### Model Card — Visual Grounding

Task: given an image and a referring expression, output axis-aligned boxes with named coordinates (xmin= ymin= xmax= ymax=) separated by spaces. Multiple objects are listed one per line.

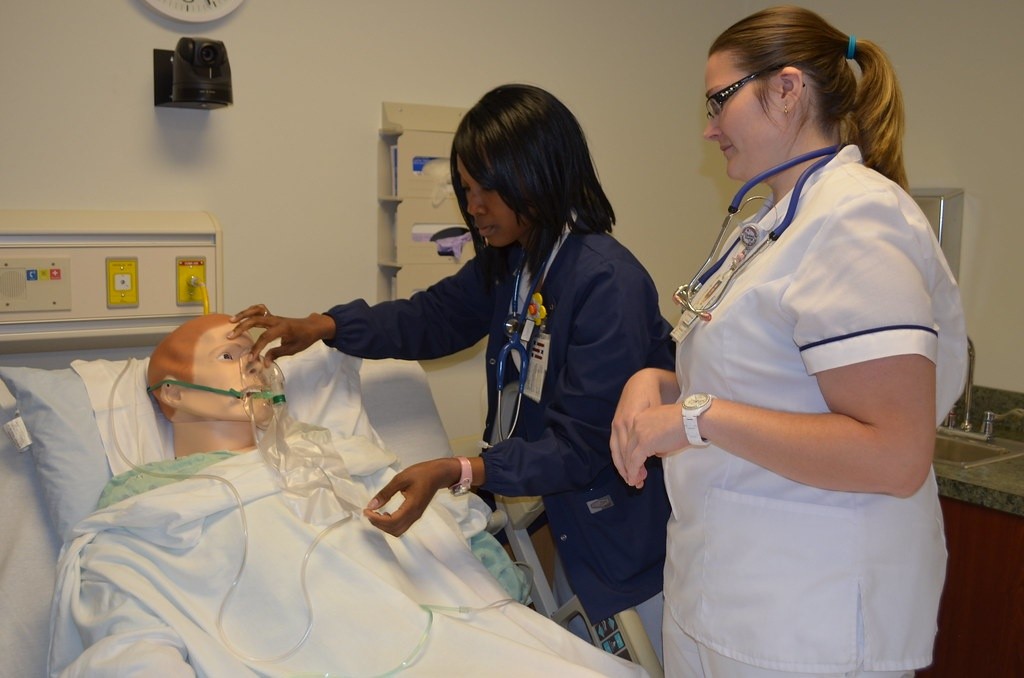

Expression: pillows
xmin=0 ymin=335 xmax=397 ymax=543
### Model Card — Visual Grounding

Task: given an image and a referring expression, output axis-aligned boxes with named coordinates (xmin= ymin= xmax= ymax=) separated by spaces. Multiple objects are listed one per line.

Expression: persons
xmin=226 ymin=84 xmax=675 ymax=678
xmin=94 ymin=314 xmax=531 ymax=610
xmin=609 ymin=5 xmax=969 ymax=678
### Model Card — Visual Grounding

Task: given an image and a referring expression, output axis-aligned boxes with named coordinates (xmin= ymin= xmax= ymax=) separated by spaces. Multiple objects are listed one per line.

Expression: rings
xmin=264 ymin=311 xmax=268 ymax=316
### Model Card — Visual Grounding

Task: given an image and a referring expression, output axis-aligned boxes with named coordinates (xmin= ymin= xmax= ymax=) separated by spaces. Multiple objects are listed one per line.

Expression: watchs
xmin=682 ymin=393 xmax=718 ymax=446
xmin=448 ymin=456 xmax=473 ymax=496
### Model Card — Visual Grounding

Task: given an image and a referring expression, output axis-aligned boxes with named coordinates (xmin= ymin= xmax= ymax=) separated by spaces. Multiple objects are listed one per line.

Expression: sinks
xmin=931 ymin=429 xmax=1024 ymax=469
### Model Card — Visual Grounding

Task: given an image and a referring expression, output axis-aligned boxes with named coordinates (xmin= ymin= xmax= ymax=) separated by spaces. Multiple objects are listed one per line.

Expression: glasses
xmin=706 ymin=61 xmax=808 ymax=121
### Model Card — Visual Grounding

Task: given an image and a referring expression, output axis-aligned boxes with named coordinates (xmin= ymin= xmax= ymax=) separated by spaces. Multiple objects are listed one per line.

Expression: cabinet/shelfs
xmin=377 ymin=101 xmax=487 ymax=301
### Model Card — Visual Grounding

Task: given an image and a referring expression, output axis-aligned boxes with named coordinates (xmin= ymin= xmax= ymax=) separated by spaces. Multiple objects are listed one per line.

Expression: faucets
xmin=959 ymin=334 xmax=975 ymax=431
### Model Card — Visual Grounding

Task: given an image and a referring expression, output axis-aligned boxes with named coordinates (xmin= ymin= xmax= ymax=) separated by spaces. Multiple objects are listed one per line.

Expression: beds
xmin=0 ymin=335 xmax=664 ymax=678
